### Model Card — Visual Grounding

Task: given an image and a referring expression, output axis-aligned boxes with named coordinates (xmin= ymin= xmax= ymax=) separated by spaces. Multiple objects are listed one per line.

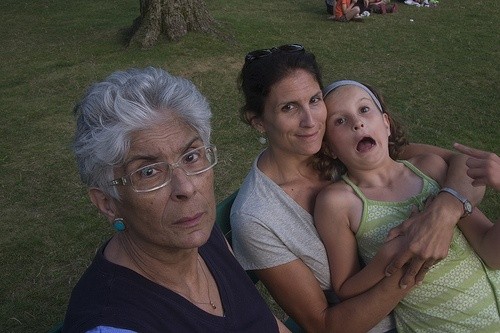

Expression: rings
xmin=422 ymin=264 xmax=432 ymax=272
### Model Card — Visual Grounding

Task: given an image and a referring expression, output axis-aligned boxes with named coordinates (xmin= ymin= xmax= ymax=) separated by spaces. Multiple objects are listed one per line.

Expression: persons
xmin=314 ymin=79 xmax=500 ymax=333
xmin=54 ymin=66 xmax=292 ymax=333
xmin=335 ymin=0 xmax=396 ymax=23
xmin=230 ymin=43 xmax=487 ymax=333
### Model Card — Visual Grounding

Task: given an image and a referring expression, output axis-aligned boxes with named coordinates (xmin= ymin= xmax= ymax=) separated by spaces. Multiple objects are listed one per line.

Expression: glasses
xmin=107 ymin=145 xmax=218 ymax=193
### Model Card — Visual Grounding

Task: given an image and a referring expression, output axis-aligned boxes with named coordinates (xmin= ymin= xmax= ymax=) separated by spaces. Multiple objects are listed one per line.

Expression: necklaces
xmin=153 ymin=255 xmax=217 ymax=310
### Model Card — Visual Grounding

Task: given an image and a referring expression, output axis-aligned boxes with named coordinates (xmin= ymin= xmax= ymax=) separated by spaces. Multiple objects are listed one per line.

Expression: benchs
xmin=216 ymin=189 xmax=305 ymax=333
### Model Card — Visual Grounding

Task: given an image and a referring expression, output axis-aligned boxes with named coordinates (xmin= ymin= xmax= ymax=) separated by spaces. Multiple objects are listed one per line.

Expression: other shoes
xmin=361 ymin=11 xmax=370 ymax=16
xmin=381 ymin=3 xmax=387 ymax=14
xmin=392 ymin=4 xmax=398 ymax=13
xmin=354 ymin=15 xmax=362 ymax=22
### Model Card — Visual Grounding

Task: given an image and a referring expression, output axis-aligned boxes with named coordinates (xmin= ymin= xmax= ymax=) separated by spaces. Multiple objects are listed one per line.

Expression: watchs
xmin=440 ymin=187 xmax=473 ymax=221
